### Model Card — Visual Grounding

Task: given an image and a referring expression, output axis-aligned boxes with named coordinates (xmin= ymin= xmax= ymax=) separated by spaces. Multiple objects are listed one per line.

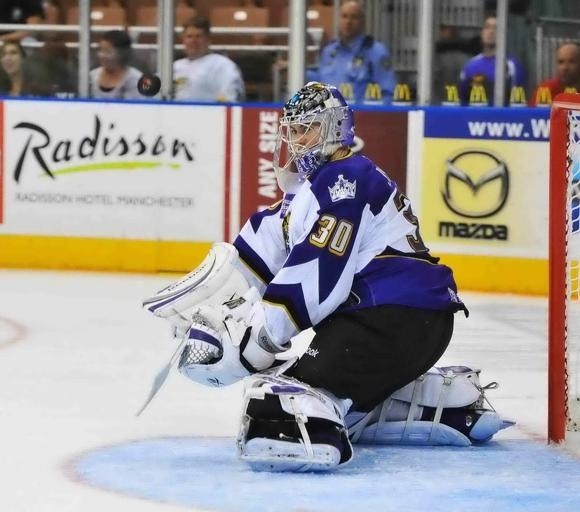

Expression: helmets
xmin=272 ymin=80 xmax=356 ymax=195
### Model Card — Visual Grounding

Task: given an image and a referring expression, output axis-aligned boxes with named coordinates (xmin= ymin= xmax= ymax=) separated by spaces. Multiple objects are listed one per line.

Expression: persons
xmin=279 ymin=1 xmax=400 ymax=100
xmin=2 ymin=1 xmax=248 ymax=103
xmin=526 ymin=43 xmax=580 ymax=106
xmin=139 ymin=79 xmax=508 ymax=475
xmin=457 ymin=18 xmax=525 ymax=102
